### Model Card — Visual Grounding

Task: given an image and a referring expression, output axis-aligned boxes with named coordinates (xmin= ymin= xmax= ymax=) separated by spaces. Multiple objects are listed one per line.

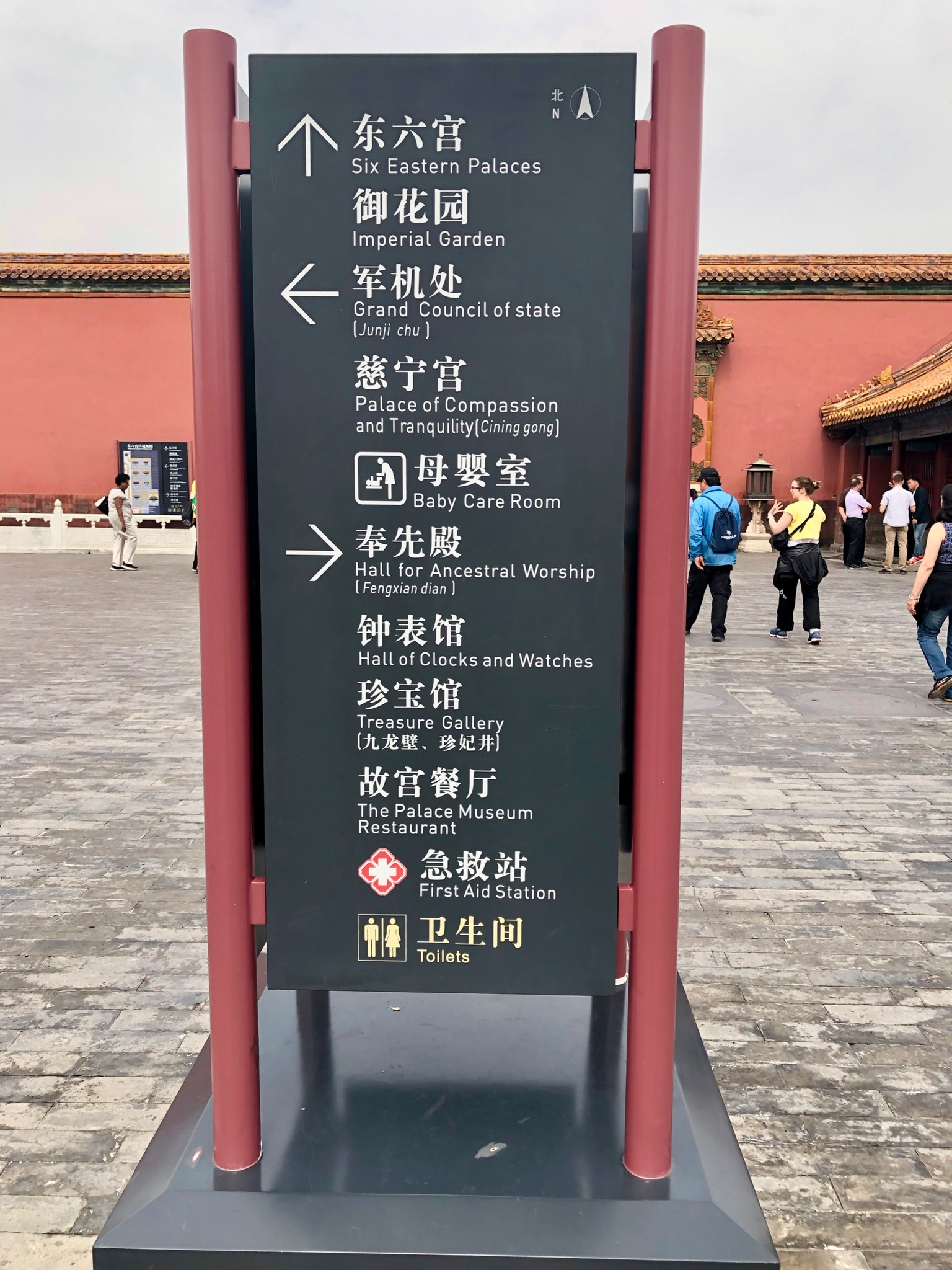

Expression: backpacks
xmin=182 ymin=499 xmax=194 ymax=526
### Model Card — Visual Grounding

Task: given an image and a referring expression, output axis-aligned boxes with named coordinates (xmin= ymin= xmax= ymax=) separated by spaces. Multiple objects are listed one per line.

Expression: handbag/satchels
xmin=94 ymin=494 xmax=109 ymax=516
xmin=697 ymin=494 xmax=739 ymax=554
xmin=773 ymin=527 xmax=790 ymax=552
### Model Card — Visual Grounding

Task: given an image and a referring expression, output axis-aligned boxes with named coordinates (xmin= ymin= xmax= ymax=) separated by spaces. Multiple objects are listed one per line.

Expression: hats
xmin=695 ymin=467 xmax=719 ymax=483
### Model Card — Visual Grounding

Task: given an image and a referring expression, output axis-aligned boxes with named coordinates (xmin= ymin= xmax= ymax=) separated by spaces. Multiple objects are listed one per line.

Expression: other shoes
xmin=879 ymin=569 xmax=891 ymax=574
xmin=685 ymin=630 xmax=691 ymax=635
xmin=192 ymin=568 xmax=196 ymax=571
xmin=900 ymin=570 xmax=907 ymax=575
xmin=860 ymin=561 xmax=868 ymax=569
xmin=712 ymin=634 xmax=725 ymax=641
xmin=850 ymin=563 xmax=862 ymax=570
xmin=846 ymin=565 xmax=850 ymax=569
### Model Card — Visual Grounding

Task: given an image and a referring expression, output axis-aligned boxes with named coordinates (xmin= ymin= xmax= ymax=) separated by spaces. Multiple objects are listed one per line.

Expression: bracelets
xmin=767 ymin=516 xmax=775 ymax=519
xmin=908 ymin=595 xmax=920 ymax=600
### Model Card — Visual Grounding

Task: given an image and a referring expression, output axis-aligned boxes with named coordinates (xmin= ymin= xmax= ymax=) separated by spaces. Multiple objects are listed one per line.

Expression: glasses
xmin=788 ymin=485 xmax=802 ymax=489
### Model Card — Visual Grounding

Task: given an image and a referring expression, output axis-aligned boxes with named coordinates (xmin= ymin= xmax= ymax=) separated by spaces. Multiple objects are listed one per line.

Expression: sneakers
xmin=110 ymin=565 xmax=126 ymax=570
xmin=769 ymin=627 xmax=788 ymax=639
xmin=928 ymin=675 xmax=952 ymax=699
xmin=906 ymin=556 xmax=921 ymax=566
xmin=918 ymin=554 xmax=924 ymax=560
xmin=941 ymin=694 xmax=952 ymax=702
xmin=121 ymin=562 xmax=138 ymax=570
xmin=807 ymin=632 xmax=822 ymax=644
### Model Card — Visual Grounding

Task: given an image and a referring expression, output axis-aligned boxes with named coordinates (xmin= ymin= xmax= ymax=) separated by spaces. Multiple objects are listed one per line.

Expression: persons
xmin=907 ymin=484 xmax=952 ymax=702
xmin=906 ymin=476 xmax=931 ymax=564
xmin=845 ymin=478 xmax=872 ymax=569
xmin=108 ymin=473 xmax=138 ymax=571
xmin=688 ymin=488 xmax=698 ymax=520
xmin=838 ymin=474 xmax=868 ymax=566
xmin=881 ymin=471 xmax=914 ymax=561
xmin=767 ymin=476 xmax=826 ymax=644
xmin=685 ymin=466 xmax=741 ymax=642
xmin=189 ymin=479 xmax=198 ymax=574
xmin=878 ymin=474 xmax=916 ymax=574
